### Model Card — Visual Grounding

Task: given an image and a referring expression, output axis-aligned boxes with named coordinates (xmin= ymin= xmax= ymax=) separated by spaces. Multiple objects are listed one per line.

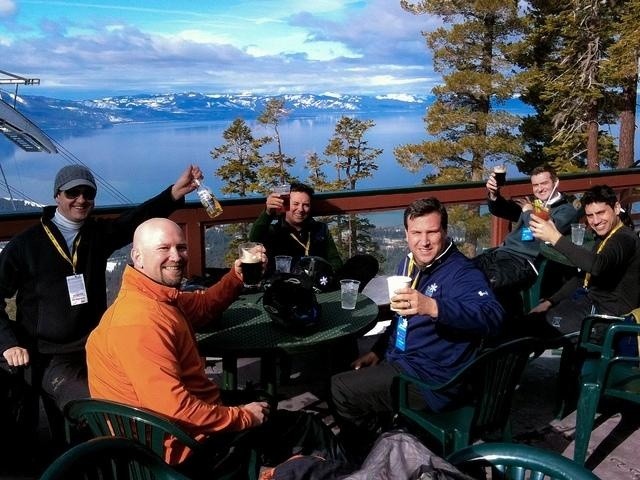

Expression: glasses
xmin=64 ymin=188 xmax=95 ymax=200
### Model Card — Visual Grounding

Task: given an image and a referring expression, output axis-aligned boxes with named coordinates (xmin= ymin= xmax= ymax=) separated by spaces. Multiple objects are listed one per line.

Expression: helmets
xmin=263 ymin=277 xmax=322 ymax=336
xmin=292 ymin=255 xmax=338 ymax=294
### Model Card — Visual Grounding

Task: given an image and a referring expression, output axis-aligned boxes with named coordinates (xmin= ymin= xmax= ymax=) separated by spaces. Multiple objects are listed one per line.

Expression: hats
xmin=54 ymin=164 xmax=97 ymax=198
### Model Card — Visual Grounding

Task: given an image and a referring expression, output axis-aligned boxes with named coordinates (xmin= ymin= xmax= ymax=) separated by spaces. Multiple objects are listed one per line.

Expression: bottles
xmin=190 ymin=171 xmax=223 ymax=218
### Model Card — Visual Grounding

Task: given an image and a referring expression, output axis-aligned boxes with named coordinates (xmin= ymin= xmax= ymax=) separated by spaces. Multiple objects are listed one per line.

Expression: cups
xmin=494 ymin=166 xmax=506 ymax=186
xmin=275 ymin=256 xmax=292 ymax=273
xmin=238 ymin=243 xmax=262 ymax=288
xmin=387 ymin=276 xmax=412 ymax=311
xmin=340 ymin=279 xmax=360 ymax=309
xmin=532 ymin=204 xmax=550 ymax=222
xmin=274 ymin=185 xmax=291 ymax=212
xmin=571 ymin=224 xmax=586 ymax=246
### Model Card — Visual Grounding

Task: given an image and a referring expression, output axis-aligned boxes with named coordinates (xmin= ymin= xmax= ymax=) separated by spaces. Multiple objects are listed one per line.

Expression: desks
xmin=193 ymin=290 xmax=379 ymax=395
xmin=540 ymin=231 xmax=595 ymax=268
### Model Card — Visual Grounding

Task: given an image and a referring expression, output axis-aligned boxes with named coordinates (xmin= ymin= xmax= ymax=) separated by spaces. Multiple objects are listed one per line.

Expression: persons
xmin=83 ymin=216 xmax=336 ymax=480
xmin=331 ymin=196 xmax=507 ymax=472
xmin=249 ymin=181 xmax=379 ymax=294
xmin=527 ymin=184 xmax=639 ymax=350
xmin=1 ymin=163 xmax=206 ymax=422
xmin=466 ymin=162 xmax=586 ymax=300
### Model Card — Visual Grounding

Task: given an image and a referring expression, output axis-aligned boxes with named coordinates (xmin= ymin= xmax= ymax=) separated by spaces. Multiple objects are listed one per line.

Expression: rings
xmin=406 ymin=301 xmax=411 ymax=308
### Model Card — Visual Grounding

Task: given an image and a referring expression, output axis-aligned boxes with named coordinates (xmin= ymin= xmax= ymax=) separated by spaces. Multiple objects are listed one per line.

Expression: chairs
xmin=40 ymin=436 xmax=188 ymax=480
xmin=445 ymin=443 xmax=600 ymax=480
xmin=62 ymin=395 xmax=278 ymax=480
xmin=556 ymin=313 xmax=640 ymax=467
xmin=395 ymin=335 xmax=538 ymax=456
xmin=523 ymin=259 xmax=550 ymax=315
